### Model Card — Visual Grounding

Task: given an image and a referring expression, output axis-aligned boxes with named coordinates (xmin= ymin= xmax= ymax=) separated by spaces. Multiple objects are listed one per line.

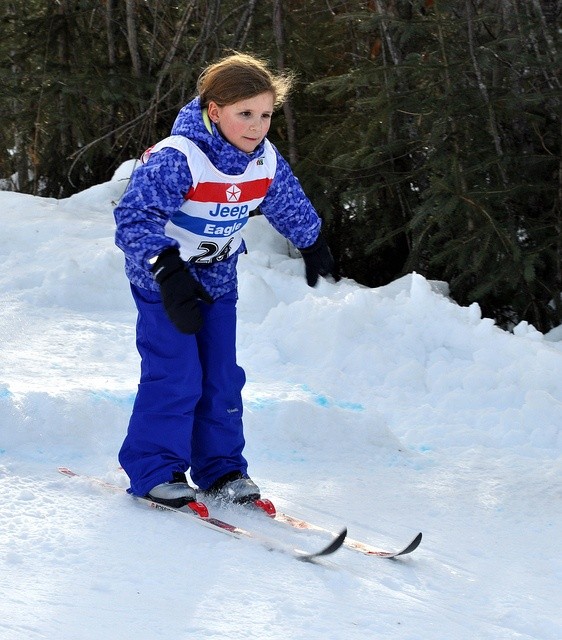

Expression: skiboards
xmin=59 ymin=465 xmax=423 ymax=561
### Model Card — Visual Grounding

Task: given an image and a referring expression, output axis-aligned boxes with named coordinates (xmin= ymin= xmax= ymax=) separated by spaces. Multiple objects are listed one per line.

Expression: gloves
xmin=297 ymin=233 xmax=342 ymax=286
xmin=151 ymin=247 xmax=215 ymax=334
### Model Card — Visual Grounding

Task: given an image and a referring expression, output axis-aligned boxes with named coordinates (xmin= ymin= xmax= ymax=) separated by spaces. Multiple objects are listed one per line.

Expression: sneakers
xmin=148 ymin=472 xmax=196 ymax=508
xmin=208 ymin=470 xmax=260 ymax=505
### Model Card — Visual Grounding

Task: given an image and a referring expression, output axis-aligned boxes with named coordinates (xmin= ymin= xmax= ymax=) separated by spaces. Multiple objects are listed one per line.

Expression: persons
xmin=112 ymin=44 xmax=332 ymax=512
